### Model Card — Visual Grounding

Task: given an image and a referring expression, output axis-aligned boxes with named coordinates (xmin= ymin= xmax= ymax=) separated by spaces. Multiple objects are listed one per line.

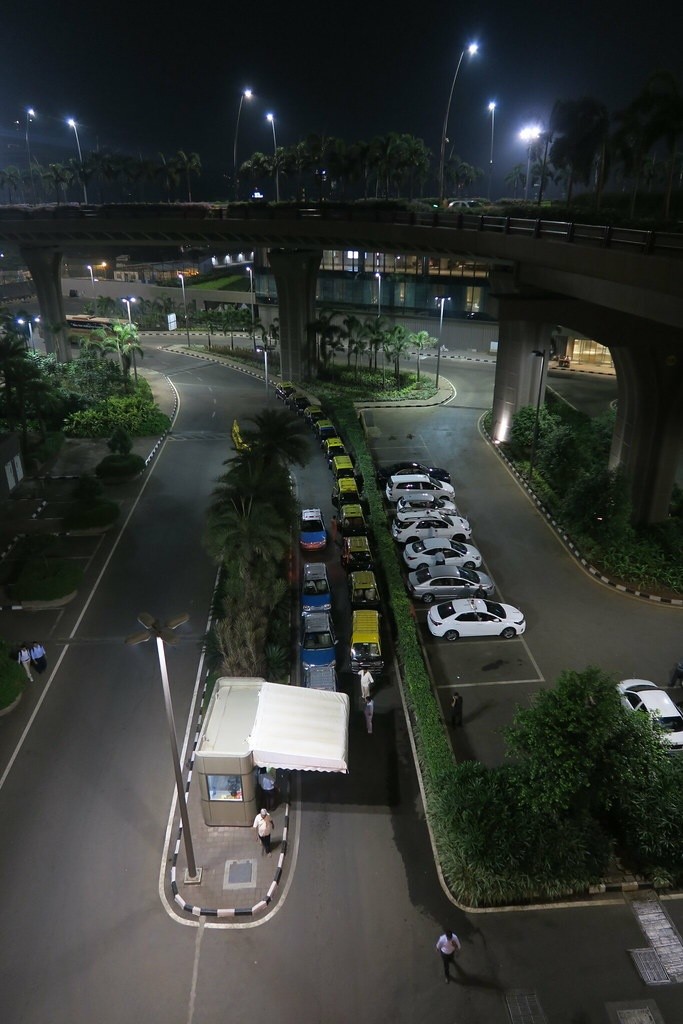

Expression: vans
xmin=299 ymin=561 xmax=333 ymax=614
xmin=274 ymin=378 xmax=388 ymax=612
xmin=298 ymin=608 xmax=340 ymax=670
xmin=302 ymin=663 xmax=339 ymax=692
xmin=346 ymin=608 xmax=390 ymax=672
xmin=297 ymin=507 xmax=329 ymax=553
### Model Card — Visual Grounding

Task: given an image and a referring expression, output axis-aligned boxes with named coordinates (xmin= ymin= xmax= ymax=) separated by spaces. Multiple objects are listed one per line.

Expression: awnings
xmin=250 ymin=682 xmax=350 ymax=773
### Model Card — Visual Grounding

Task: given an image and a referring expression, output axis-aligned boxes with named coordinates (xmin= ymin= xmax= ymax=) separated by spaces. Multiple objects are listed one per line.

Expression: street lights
xmin=233 ymin=88 xmax=253 ymax=200
xmin=125 ymin=605 xmax=203 ymax=884
xmin=121 ymin=296 xmax=139 ymax=383
xmin=527 ymin=348 xmax=546 ymax=481
xmin=87 ymin=265 xmax=95 ymax=283
xmin=488 ymin=102 xmax=496 ymax=205
xmin=266 ymin=112 xmax=278 ymax=203
xmin=18 ymin=317 xmax=41 ymax=355
xmin=440 ymin=40 xmax=479 ymax=206
xmin=177 ymin=272 xmax=190 ymax=345
xmin=521 ymin=127 xmax=543 ymax=202
xmin=375 ymin=272 xmax=381 ymax=318
xmin=25 ymin=108 xmax=37 ymax=208
xmin=66 ymin=118 xmax=87 ymax=206
xmin=256 ymin=348 xmax=269 ymax=390
xmin=433 ymin=297 xmax=451 ymax=387
xmin=245 ymin=267 xmax=257 ymax=351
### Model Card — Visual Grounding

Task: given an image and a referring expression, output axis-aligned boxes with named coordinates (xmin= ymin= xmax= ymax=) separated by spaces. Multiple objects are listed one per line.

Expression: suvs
xmin=385 ymin=474 xmax=456 ymax=502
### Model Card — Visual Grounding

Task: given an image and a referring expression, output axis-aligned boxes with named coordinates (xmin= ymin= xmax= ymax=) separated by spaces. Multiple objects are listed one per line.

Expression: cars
xmin=377 ymin=462 xmax=452 ymax=491
xmin=407 ymin=564 xmax=497 ymax=605
xmin=448 ymin=200 xmax=482 ymax=208
xmin=403 ymin=537 xmax=486 ymax=570
xmin=614 ymin=678 xmax=683 ymax=757
xmin=392 ymin=510 xmax=473 ymax=543
xmin=427 ymin=597 xmax=526 ymax=642
xmin=395 ymin=492 xmax=458 ymax=516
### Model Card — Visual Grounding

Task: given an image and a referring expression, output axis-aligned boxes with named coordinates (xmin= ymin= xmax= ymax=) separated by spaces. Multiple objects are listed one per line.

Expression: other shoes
xmin=445 ymin=975 xmax=450 ymax=984
xmin=267 ymin=852 xmax=272 ymax=857
xmin=27 ymin=674 xmax=33 ymax=682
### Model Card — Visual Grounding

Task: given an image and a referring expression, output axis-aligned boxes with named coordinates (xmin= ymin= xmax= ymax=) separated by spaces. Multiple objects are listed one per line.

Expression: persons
xmin=18 ymin=642 xmax=47 ymax=682
xmin=450 ymin=692 xmax=463 ymax=729
xmin=259 ymin=767 xmax=276 ymax=809
xmin=358 ymin=668 xmax=374 ymax=698
xmin=253 ymin=808 xmax=274 ymax=856
xmin=436 ymin=931 xmax=460 ymax=984
xmin=365 ymin=696 xmax=374 ymax=734
xmin=331 ymin=515 xmax=337 ymax=541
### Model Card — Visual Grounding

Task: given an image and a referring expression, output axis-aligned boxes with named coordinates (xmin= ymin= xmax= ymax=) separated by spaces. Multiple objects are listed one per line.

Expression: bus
xmin=38 ymin=314 xmax=139 ymax=353
